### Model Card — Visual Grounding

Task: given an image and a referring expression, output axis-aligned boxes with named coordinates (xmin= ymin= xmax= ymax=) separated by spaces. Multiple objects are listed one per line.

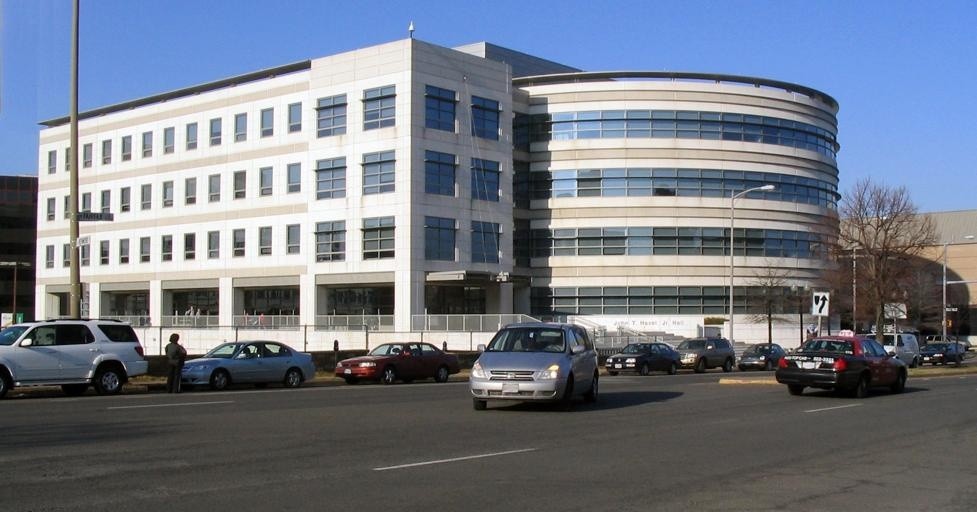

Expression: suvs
xmin=675 ymin=337 xmax=736 ymax=373
xmin=0 ymin=317 xmax=151 ymax=399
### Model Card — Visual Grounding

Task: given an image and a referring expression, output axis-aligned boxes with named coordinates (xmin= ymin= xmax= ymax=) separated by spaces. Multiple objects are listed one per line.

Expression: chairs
xmin=825 ymin=346 xmax=837 ymax=351
xmin=411 ymin=348 xmax=418 ymax=356
xmin=243 ymin=348 xmax=251 ymax=354
xmin=392 ymin=348 xmax=401 ymax=355
xmin=512 ymin=338 xmax=528 ymax=349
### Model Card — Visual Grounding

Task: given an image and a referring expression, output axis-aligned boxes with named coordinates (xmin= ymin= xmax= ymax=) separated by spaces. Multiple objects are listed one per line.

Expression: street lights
xmin=940 ymin=234 xmax=974 ymax=342
xmin=727 ymin=183 xmax=777 ymax=348
xmin=850 ymin=215 xmax=888 ymax=335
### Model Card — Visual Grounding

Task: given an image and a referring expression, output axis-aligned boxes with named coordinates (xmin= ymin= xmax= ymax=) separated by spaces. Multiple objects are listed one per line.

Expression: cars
xmin=334 ymin=341 xmax=460 ymax=385
xmin=603 ymin=342 xmax=682 ymax=375
xmin=180 ymin=338 xmax=316 ymax=390
xmin=919 ymin=341 xmax=965 ymax=366
xmin=738 ymin=342 xmax=786 ymax=371
xmin=774 ymin=334 xmax=908 ymax=397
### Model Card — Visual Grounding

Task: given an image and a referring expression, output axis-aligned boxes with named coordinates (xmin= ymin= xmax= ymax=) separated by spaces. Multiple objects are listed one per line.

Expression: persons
xmin=542 ymin=332 xmax=564 ymax=352
xmin=164 ymin=333 xmax=187 ymax=393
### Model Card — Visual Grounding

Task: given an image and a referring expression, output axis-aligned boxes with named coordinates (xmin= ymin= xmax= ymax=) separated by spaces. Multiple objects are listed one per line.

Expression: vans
xmin=925 ymin=334 xmax=969 ymax=351
xmin=466 ymin=321 xmax=599 ymax=411
xmin=866 ymin=332 xmax=920 ymax=370
